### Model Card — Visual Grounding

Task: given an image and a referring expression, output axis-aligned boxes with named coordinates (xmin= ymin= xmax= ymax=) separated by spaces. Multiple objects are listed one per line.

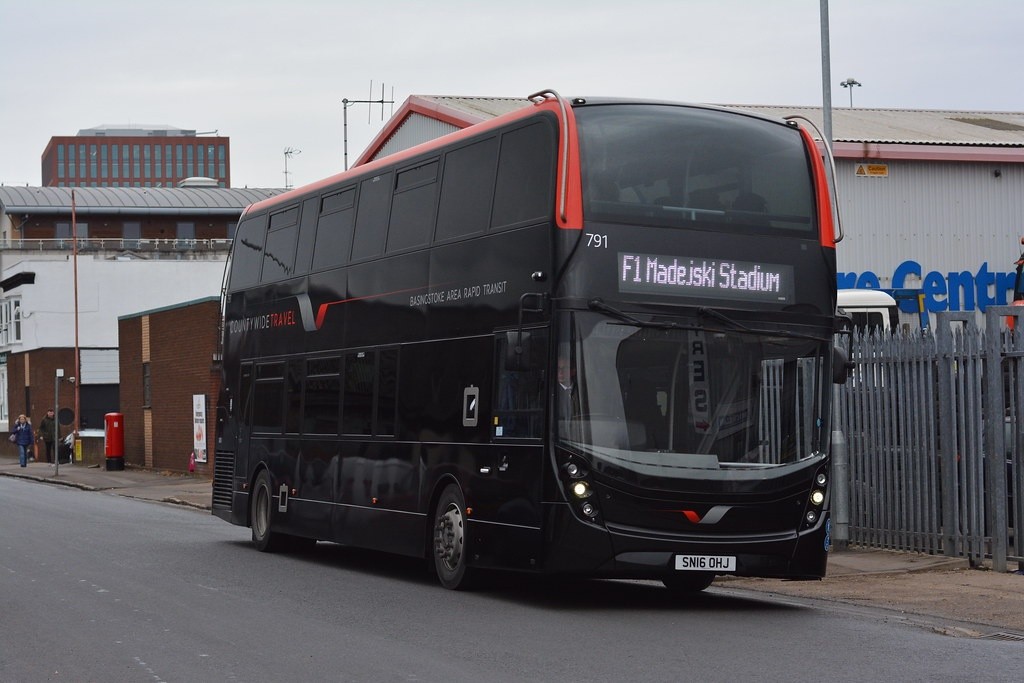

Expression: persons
xmin=39 ymin=409 xmax=63 ymax=462
xmin=11 ymin=414 xmax=34 ymax=467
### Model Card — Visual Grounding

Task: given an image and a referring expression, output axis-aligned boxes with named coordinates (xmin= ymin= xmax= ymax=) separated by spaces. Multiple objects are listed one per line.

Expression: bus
xmin=207 ymin=88 xmax=860 ymax=598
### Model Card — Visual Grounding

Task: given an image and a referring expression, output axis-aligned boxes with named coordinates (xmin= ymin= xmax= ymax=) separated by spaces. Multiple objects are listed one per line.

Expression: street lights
xmin=838 ymin=77 xmax=862 ymax=108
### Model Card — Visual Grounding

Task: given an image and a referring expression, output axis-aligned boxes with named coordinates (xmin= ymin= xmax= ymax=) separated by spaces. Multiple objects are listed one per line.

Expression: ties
xmin=566 ymin=389 xmax=572 ymax=399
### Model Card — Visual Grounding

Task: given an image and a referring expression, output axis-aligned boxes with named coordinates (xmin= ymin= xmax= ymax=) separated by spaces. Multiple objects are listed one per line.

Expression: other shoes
xmin=21 ymin=464 xmax=26 ymax=467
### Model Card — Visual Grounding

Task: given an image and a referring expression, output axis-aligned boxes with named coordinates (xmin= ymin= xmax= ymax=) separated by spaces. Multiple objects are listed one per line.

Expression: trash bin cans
xmin=105 ymin=413 xmax=125 ymax=472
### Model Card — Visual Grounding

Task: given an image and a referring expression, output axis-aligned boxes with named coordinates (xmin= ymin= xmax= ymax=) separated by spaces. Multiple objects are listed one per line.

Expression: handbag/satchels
xmin=8 ymin=433 xmax=16 ymax=443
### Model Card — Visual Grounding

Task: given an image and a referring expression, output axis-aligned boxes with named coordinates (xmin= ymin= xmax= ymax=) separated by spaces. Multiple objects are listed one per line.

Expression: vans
xmin=836 ymin=286 xmax=902 ymax=389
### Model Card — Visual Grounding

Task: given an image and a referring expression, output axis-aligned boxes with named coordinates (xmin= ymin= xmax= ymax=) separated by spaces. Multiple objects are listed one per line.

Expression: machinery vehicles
xmin=1002 ymin=238 xmax=1024 ymax=343
